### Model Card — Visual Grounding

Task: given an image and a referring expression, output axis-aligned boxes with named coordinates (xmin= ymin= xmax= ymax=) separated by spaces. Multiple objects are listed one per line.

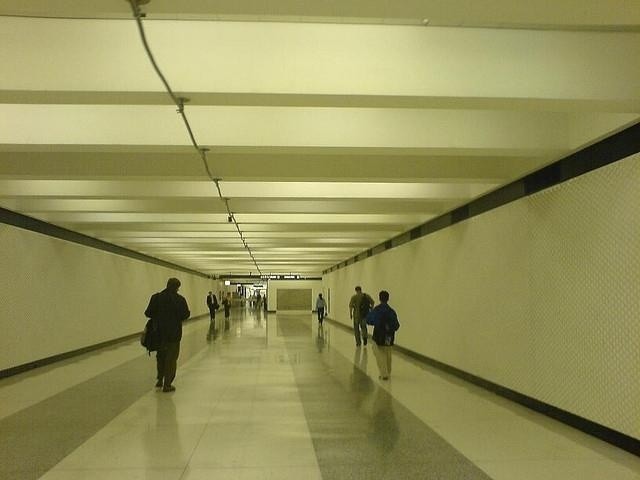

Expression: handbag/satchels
xmin=141 ymin=319 xmax=161 ymax=356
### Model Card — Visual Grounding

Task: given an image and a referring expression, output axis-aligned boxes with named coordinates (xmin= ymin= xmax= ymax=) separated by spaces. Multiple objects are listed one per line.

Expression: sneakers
xmin=156 ymin=381 xmax=175 ymax=392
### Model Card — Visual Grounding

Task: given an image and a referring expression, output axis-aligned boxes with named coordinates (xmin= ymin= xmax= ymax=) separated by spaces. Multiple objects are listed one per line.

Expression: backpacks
xmin=376 ymin=320 xmax=394 ymax=346
xmin=361 ymin=296 xmax=370 ymax=317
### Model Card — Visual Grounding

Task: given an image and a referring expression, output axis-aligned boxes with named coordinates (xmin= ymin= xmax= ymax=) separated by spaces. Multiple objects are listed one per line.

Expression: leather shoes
xmin=364 ymin=338 xmax=367 ymax=345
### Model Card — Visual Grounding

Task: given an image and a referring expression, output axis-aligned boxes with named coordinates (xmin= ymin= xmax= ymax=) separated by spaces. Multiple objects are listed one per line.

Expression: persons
xmin=315 ymin=325 xmax=326 ymax=354
xmin=365 ymin=289 xmax=401 ymax=380
xmin=315 ymin=293 xmax=328 ymax=326
xmin=145 ymin=391 xmax=191 ymax=480
xmin=366 ymin=380 xmax=401 ymax=474
xmin=222 ymin=319 xmax=231 ymax=346
xmin=349 ymin=286 xmax=375 ymax=347
xmin=144 ymin=277 xmax=190 ymax=393
xmin=239 ymin=290 xmax=267 ymax=312
xmin=344 ymin=346 xmax=376 ymax=418
xmin=222 ymin=296 xmax=231 ymax=320
xmin=206 ymin=291 xmax=218 ymax=320
xmin=206 ymin=319 xmax=219 ymax=344
